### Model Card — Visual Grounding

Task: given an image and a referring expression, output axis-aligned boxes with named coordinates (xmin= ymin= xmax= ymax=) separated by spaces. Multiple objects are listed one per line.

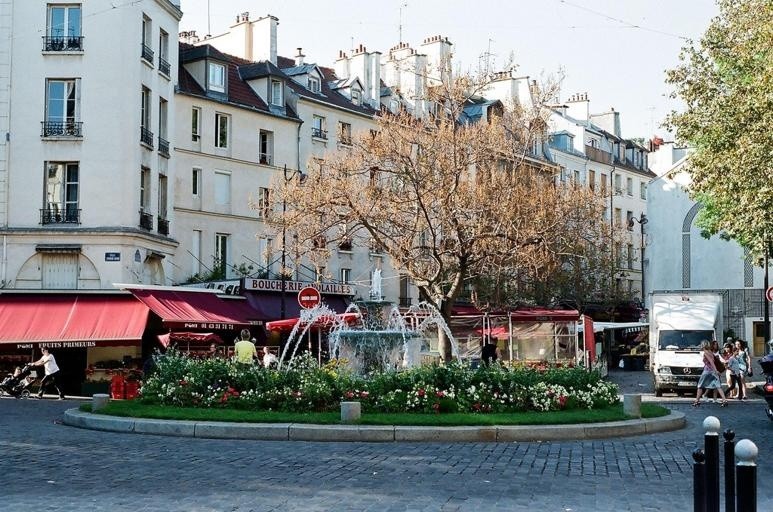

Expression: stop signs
xmin=297 ymin=287 xmax=320 ymax=311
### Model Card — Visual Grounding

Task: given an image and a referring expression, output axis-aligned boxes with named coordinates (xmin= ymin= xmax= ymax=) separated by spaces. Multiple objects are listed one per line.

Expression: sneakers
xmin=59 ymin=398 xmax=65 ymax=400
xmin=35 ymin=395 xmax=42 ymax=399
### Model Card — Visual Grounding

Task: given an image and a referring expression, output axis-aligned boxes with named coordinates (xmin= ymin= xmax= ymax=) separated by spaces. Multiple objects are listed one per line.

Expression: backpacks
xmin=747 ymin=367 xmax=753 ymax=377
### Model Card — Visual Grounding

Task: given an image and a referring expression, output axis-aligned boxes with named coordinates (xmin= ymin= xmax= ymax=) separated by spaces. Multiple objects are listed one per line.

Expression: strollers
xmin=0 ymin=363 xmax=36 ymax=400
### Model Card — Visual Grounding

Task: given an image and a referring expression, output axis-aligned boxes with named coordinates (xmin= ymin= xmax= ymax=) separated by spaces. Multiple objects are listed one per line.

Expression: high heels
xmin=720 ymin=400 xmax=728 ymax=408
xmin=692 ymin=402 xmax=701 ymax=407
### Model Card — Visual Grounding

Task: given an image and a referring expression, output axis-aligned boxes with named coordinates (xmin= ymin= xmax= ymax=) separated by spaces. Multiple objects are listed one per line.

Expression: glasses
xmin=41 ymin=349 xmax=45 ymax=352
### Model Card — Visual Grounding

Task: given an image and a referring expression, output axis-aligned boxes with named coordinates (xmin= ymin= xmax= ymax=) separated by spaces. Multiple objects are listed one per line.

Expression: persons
xmin=233 ymin=329 xmax=261 ymax=371
xmin=481 ymin=336 xmax=502 ymax=369
xmin=1 ymin=366 xmax=21 ymax=385
xmin=262 ymin=346 xmax=279 ymax=369
xmin=26 ymin=346 xmax=64 ymax=400
xmin=690 ymin=336 xmax=753 ymax=407
xmin=205 ymin=342 xmax=219 ymax=361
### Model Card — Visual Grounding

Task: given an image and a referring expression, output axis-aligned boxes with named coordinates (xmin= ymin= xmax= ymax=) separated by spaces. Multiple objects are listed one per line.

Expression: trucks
xmin=648 ymin=293 xmax=723 ymax=398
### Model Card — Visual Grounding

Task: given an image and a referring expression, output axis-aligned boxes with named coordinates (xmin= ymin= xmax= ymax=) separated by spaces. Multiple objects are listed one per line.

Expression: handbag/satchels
xmin=712 ymin=354 xmax=726 ymax=373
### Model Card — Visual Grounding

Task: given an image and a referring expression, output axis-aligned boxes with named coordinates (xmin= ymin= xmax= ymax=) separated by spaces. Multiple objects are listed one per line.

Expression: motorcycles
xmin=759 ymin=340 xmax=772 ymax=421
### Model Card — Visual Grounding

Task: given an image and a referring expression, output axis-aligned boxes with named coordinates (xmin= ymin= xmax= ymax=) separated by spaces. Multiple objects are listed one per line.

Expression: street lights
xmin=627 ymin=211 xmax=648 ymax=311
